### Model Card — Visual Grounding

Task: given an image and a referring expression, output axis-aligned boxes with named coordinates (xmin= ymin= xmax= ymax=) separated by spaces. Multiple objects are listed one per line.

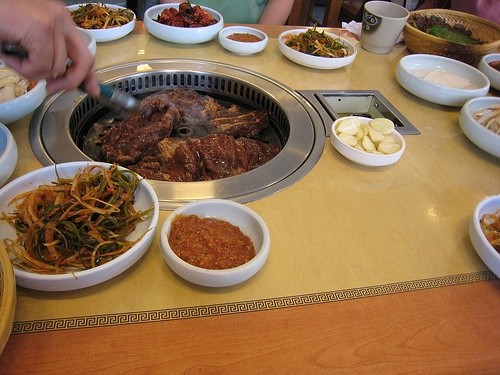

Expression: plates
xmin=329 ymin=116 xmax=406 ymax=167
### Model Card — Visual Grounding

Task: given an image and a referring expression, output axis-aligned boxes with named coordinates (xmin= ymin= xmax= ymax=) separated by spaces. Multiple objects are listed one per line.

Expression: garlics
xmin=335 ymin=117 xmax=402 ymax=155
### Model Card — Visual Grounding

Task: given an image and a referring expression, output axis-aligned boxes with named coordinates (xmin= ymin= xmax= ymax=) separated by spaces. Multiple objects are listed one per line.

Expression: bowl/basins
xmin=160 ymin=198 xmax=271 ymax=288
xmin=65 ymin=26 xmax=97 ymax=68
xmin=395 ymin=53 xmax=491 ymax=107
xmin=478 ymin=52 xmax=500 ymax=91
xmin=0 ymin=123 xmax=19 ymax=187
xmin=277 ymin=29 xmax=357 ymax=70
xmin=0 ymin=61 xmax=47 ymax=126
xmin=65 ymin=3 xmax=136 ymax=43
xmin=144 ymin=3 xmax=224 ymax=44
xmin=216 ymin=25 xmax=269 ymax=56
xmin=0 ymin=160 xmax=160 ymax=292
xmin=468 ymin=194 xmax=500 ymax=279
xmin=459 ymin=96 xmax=500 ymax=159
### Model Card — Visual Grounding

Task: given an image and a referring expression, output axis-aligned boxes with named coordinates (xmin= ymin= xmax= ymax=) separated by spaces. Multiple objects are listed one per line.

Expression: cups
xmin=360 ymin=1 xmax=410 ymax=55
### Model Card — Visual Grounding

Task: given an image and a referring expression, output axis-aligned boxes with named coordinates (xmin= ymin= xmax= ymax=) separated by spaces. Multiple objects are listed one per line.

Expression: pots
xmin=40 ymin=58 xmax=315 ymax=203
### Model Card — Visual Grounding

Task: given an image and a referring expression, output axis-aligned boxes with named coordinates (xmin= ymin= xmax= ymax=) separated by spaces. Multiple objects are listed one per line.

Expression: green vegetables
xmin=14 ymin=166 xmax=151 ymax=273
xmin=285 ymin=22 xmax=348 ymax=56
xmin=409 ymin=21 xmax=486 ymax=44
xmin=65 ymin=1 xmax=134 ymax=30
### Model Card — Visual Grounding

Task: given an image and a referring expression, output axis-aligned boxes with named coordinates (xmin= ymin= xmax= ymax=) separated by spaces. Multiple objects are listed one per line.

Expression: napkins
xmin=342 ymin=20 xmax=404 ymax=44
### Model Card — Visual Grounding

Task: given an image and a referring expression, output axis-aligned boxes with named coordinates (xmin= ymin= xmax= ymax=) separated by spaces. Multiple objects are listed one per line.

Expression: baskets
xmin=403 ymin=8 xmax=500 ymax=63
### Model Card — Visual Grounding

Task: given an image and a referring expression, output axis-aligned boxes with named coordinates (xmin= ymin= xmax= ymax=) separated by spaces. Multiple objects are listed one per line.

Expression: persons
xmin=0 ymin=0 xmax=101 ymax=98
xmin=137 ymin=0 xmax=295 ymax=25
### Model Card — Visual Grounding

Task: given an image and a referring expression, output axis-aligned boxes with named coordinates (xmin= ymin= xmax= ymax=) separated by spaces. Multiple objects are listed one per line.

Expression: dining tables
xmin=0 ymin=24 xmax=500 ymax=375
